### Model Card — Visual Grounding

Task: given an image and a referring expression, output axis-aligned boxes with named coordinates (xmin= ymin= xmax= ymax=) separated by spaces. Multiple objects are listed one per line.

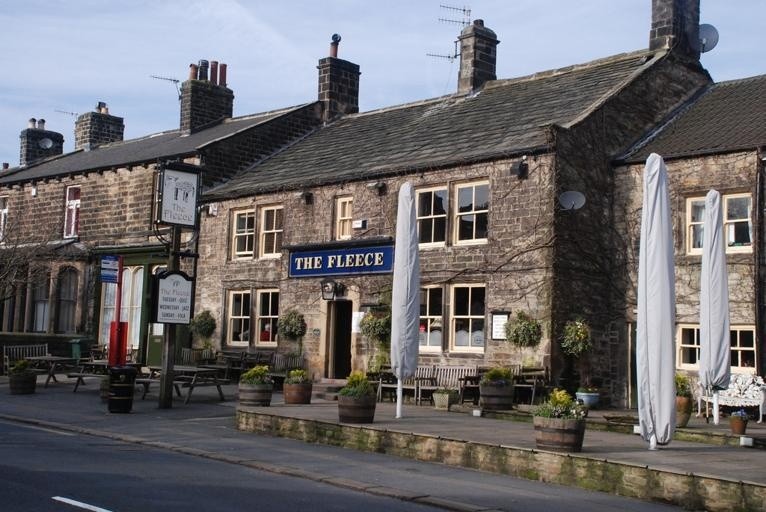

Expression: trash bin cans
xmin=68 ymin=338 xmax=93 ymax=358
xmin=108 ymin=366 xmax=137 ymax=414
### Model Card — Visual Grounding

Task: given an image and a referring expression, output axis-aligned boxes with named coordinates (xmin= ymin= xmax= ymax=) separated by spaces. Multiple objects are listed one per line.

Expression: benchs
xmin=457 ymin=369 xmax=548 ymax=406
xmin=379 ymin=364 xmax=478 ymax=407
xmin=266 ymin=353 xmax=305 ymax=391
xmin=2 ymin=343 xmax=273 ymax=406
xmin=694 ymin=373 xmax=766 ymax=424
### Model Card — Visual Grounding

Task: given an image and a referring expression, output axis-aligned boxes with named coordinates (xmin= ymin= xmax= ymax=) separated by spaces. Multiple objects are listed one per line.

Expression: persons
xmin=260 ymin=322 xmax=271 ymax=341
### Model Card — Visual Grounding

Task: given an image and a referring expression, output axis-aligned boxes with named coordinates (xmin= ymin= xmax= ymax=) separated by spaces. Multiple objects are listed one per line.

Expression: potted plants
xmin=727 ymin=413 xmax=753 ymax=435
xmin=575 ymin=387 xmax=600 ymax=410
xmin=674 ymin=372 xmax=693 ymax=428
xmin=532 ymin=388 xmax=587 ymax=453
xmin=478 ymin=367 xmax=515 ymax=410
xmin=337 ymin=368 xmax=376 ymax=423
xmin=282 ymin=368 xmax=314 ymax=404
